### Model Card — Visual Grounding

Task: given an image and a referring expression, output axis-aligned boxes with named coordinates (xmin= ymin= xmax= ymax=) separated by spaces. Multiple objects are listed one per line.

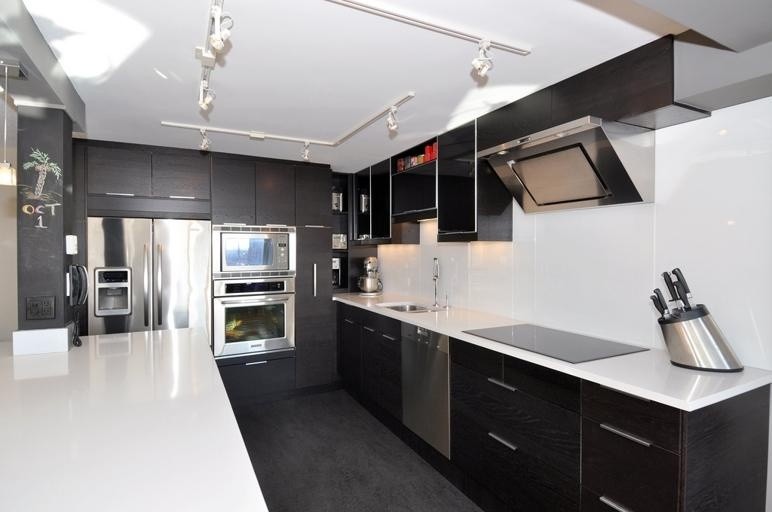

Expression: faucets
xmin=432 ymin=277 xmax=440 ymax=307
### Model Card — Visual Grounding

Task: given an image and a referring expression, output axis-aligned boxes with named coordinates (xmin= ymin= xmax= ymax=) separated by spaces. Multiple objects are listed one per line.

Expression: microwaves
xmin=212 ymin=224 xmax=290 ymax=273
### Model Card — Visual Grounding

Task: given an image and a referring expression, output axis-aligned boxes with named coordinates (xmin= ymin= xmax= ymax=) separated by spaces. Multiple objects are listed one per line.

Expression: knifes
xmin=650 ymin=268 xmax=696 ymax=320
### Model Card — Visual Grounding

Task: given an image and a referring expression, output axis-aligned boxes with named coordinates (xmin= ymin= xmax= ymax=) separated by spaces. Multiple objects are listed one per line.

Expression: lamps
xmin=472 ymin=39 xmax=494 ymax=77
xmin=383 ymin=105 xmax=400 ymax=135
xmin=197 ymin=5 xmax=235 ymax=115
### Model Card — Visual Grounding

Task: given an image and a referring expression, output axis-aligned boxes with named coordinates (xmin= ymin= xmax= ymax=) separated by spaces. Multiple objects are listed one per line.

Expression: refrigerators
xmin=85 ymin=216 xmax=212 ymax=350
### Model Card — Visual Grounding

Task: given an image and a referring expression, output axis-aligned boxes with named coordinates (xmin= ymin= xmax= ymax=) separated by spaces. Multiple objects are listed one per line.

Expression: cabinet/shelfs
xmin=86 ymin=147 xmax=211 ymax=220
xmin=214 ymin=156 xmax=333 ymax=229
xmin=217 ymin=357 xmax=295 ymax=399
xmin=295 ymin=228 xmax=338 ymax=389
xmin=449 ymin=359 xmax=770 ymax=512
xmin=338 ymin=307 xmax=400 ymax=421
xmin=476 ymin=33 xmax=711 ymax=152
xmin=333 ymin=118 xmax=513 ymax=244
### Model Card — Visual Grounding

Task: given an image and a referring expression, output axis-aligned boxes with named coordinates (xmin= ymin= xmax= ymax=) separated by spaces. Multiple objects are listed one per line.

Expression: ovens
xmin=214 ymin=279 xmax=296 ymax=357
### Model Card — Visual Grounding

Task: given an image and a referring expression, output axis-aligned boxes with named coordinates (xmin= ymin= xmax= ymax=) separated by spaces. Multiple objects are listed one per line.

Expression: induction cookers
xmin=463 ymin=324 xmax=651 ymax=363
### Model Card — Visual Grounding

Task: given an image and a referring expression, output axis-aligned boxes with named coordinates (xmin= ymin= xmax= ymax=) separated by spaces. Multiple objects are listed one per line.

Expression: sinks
xmin=385 ymin=305 xmax=428 ymax=313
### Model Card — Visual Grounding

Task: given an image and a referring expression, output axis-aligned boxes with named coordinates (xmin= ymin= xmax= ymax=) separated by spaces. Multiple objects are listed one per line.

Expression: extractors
xmin=477 ymin=115 xmax=656 ymax=215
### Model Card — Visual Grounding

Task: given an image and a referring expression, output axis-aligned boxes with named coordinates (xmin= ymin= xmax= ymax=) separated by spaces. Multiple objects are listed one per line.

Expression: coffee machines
xmin=358 ymin=256 xmax=383 ymax=296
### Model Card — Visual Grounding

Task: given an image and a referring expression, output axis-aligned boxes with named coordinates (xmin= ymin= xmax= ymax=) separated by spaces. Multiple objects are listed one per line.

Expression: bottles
xmin=396 ymin=142 xmax=438 ymax=172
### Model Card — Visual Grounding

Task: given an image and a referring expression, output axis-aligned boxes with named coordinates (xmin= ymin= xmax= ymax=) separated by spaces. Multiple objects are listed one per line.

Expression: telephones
xmin=69 ymin=264 xmax=88 ymax=307
